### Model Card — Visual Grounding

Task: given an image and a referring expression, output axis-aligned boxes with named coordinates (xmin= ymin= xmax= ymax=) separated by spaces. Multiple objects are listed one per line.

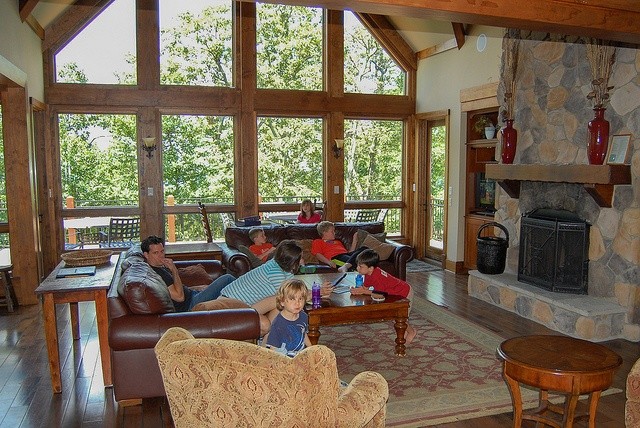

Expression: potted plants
xmin=475 ymin=118 xmax=497 ymax=142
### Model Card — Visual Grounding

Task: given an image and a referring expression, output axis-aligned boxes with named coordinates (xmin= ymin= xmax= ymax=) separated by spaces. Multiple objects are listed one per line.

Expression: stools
xmin=0 ymin=265 xmax=17 ymax=311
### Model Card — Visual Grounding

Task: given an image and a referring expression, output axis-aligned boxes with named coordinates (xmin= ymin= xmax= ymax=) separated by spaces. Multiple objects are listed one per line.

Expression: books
xmin=331 ymin=282 xmax=350 ymax=294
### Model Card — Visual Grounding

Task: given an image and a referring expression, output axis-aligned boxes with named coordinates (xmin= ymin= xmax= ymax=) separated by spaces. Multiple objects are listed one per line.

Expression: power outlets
xmin=412 ymin=183 xmax=415 ymax=192
xmin=448 ymin=198 xmax=452 ymax=207
xmin=448 ymin=187 xmax=453 ymax=195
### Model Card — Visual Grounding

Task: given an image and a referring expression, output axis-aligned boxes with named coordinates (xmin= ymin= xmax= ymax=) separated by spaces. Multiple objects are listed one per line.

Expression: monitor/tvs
xmin=476 ymin=179 xmax=497 ymax=214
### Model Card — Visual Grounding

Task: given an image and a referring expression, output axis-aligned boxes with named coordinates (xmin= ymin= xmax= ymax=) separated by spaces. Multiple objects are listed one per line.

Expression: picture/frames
xmin=606 ymin=132 xmax=632 ymax=165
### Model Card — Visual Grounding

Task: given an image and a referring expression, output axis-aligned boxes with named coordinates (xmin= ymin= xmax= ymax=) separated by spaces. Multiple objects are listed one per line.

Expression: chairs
xmin=146 ymin=185 xmax=155 ymax=198
xmin=219 ymin=211 xmax=231 ymax=234
xmin=264 ymin=212 xmax=325 ymax=223
xmin=141 ymin=136 xmax=157 ymax=158
xmin=197 ymin=202 xmax=212 ymax=243
xmin=333 ymin=186 xmax=340 ymax=196
xmin=64 ymin=228 xmax=82 ymax=249
xmin=314 ymin=201 xmax=326 ymax=223
xmin=100 ymin=218 xmax=141 ymax=244
xmin=377 ymin=210 xmax=388 ymax=222
xmin=357 ymin=211 xmax=380 ymax=222
xmin=344 ymin=210 xmax=362 ymax=222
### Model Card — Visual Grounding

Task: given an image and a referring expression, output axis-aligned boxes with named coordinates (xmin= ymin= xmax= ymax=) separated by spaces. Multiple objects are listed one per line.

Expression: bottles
xmin=356 ymin=275 xmax=363 ymax=288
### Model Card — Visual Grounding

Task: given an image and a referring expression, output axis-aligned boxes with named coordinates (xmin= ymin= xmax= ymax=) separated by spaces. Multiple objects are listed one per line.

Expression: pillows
xmin=361 ymin=236 xmax=396 ymax=260
xmin=356 ymin=229 xmax=386 ymax=249
xmin=176 ymin=261 xmax=213 ymax=287
xmin=294 ymin=239 xmax=319 ymax=263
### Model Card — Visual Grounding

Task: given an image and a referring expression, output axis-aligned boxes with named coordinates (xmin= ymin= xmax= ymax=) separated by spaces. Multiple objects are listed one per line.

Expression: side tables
xmin=495 ymin=336 xmax=622 ymax=428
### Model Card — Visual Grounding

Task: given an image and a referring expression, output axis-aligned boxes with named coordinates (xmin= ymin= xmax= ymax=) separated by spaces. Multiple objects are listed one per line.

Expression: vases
xmin=500 ymin=120 xmax=519 ymax=163
xmin=587 ymin=106 xmax=609 ymax=164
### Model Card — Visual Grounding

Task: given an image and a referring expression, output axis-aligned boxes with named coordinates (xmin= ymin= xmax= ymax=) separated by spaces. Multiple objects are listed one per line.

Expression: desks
xmin=64 ymin=220 xmax=102 ymax=248
xmin=33 ymin=249 xmax=119 ymax=396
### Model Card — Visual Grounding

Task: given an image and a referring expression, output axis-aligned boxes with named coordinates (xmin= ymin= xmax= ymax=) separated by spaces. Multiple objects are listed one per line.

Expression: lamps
xmin=332 ymin=138 xmax=344 ymax=158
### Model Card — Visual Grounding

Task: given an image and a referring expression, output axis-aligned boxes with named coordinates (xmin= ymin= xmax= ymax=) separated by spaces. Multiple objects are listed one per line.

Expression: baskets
xmin=60 ymin=249 xmax=115 ymax=266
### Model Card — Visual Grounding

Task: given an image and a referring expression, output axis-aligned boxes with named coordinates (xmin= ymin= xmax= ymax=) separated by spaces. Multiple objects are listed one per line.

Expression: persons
xmin=248 ymin=228 xmax=277 ymax=263
xmin=349 ymin=249 xmax=417 ymax=346
xmin=141 ymin=235 xmax=236 ymax=312
xmin=311 ymin=221 xmax=369 ymax=273
xmin=264 ymin=279 xmax=313 ymax=358
xmin=296 ymin=199 xmax=321 ymax=224
xmin=219 ymin=239 xmax=337 ymax=338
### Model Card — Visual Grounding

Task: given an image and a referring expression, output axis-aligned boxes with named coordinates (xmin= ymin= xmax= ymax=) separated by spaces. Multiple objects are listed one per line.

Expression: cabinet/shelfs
xmin=481 ymin=155 xmax=634 ymax=207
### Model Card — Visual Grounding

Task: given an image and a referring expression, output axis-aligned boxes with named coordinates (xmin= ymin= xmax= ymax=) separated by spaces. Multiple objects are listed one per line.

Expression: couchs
xmin=218 ymin=223 xmax=414 ymax=283
xmin=156 ymin=327 xmax=388 ymax=428
xmin=106 ymin=247 xmax=259 ymax=409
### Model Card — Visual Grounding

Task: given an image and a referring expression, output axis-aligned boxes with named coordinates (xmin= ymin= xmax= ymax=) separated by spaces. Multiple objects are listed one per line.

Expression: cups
xmin=312 ymin=281 xmax=321 ymax=305
